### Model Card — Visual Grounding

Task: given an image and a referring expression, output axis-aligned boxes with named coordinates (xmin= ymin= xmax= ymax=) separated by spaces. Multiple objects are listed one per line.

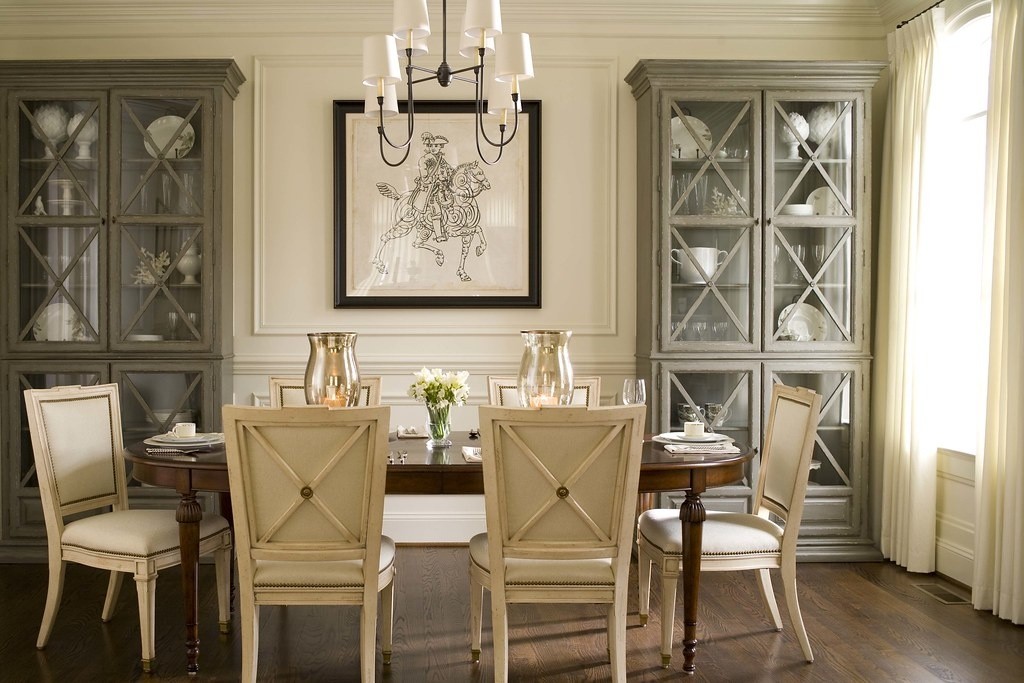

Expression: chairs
xmin=23 ymin=382 xmax=234 ymax=673
xmin=220 ymin=404 xmax=392 ymax=682
xmin=464 ymin=403 xmax=646 ymax=682
xmin=487 ymin=374 xmax=597 ymax=412
xmin=636 ymin=382 xmax=823 ymax=667
xmin=267 ymin=376 xmax=383 ymax=406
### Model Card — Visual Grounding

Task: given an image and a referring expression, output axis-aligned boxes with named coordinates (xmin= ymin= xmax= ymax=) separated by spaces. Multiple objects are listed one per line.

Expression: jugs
xmin=176 ymin=237 xmax=202 ymax=284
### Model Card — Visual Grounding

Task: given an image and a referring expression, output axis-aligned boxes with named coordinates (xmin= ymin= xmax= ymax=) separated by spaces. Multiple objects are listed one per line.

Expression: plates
xmin=145 ymin=408 xmax=194 ymax=423
xmin=671 ymin=116 xmax=712 ymax=158
xmin=143 ymin=433 xmax=225 ymax=449
xmin=33 ymin=303 xmax=86 ymax=341
xmin=144 ymin=115 xmax=195 ymax=158
xmin=677 ymin=435 xmax=710 ymax=439
xmin=806 ymin=186 xmax=845 ymax=216
xmin=652 ymin=432 xmax=724 ymax=445
xmin=778 ymin=303 xmax=828 ymax=341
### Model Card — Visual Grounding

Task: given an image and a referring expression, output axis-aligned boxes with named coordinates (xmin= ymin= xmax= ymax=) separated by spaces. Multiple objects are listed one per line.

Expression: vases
xmin=423 ymin=402 xmax=453 ymax=448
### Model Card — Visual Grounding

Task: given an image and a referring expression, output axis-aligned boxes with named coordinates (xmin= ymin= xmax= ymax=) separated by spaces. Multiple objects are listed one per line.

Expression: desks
xmin=120 ymin=431 xmax=753 ymax=676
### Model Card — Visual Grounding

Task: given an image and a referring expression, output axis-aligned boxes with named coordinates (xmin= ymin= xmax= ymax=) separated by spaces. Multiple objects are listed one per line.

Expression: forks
xmin=675 ymin=445 xmax=727 ymax=450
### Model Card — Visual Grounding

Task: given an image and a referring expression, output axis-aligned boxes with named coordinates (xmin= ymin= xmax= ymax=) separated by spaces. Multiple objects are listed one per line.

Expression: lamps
xmin=360 ymin=0 xmax=534 ymax=166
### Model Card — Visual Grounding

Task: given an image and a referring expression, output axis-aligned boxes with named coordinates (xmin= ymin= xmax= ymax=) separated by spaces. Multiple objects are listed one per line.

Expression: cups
xmin=670 ymin=247 xmax=728 ymax=284
xmin=677 ymin=402 xmax=706 ymax=425
xmin=623 ymin=379 xmax=646 ymax=404
xmin=684 ymin=421 xmax=704 ymax=437
xmin=172 ymin=423 xmax=195 ymax=436
xmin=704 ymin=402 xmax=732 ymax=427
xmin=671 ymin=173 xmax=709 ymax=214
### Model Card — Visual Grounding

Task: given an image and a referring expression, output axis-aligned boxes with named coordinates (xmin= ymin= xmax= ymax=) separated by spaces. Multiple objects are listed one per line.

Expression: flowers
xmin=405 ymin=367 xmax=473 ymax=439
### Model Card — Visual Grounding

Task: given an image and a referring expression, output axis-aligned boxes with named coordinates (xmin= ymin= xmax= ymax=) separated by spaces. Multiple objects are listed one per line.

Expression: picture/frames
xmin=331 ymin=97 xmax=542 ymax=308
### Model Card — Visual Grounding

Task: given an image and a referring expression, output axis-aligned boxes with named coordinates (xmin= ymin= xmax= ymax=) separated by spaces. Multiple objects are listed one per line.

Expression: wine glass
xmin=167 ymin=312 xmax=181 ymax=340
xmin=186 ymin=312 xmax=199 ymax=340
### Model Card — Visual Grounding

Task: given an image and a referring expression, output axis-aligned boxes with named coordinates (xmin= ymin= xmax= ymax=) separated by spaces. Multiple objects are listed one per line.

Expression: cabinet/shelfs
xmin=625 ymin=58 xmax=891 ymax=565
xmin=1 ymin=57 xmax=250 ymax=559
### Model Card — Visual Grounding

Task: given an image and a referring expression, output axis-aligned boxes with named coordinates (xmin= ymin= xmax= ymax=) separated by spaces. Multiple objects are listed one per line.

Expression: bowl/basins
xmin=783 ymin=204 xmax=812 ymax=215
